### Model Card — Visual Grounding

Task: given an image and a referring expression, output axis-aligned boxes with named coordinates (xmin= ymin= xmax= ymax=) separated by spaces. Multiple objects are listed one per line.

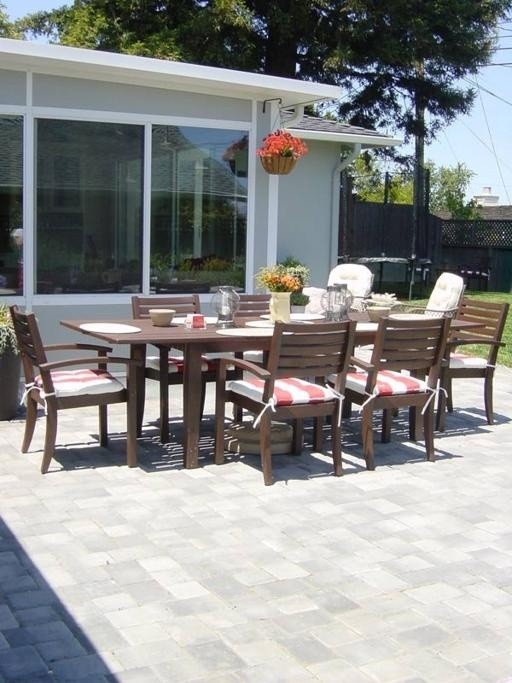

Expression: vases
xmin=268 ymin=292 xmax=293 ymax=318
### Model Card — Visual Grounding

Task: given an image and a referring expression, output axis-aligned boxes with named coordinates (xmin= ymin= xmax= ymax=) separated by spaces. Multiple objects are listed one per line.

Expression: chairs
xmin=425 ymin=297 xmax=510 ymax=433
xmin=61 ymin=281 xmax=119 ymax=293
xmin=328 ymin=313 xmax=452 ymax=471
xmin=390 ymin=272 xmax=467 ymax=320
xmin=37 ymin=281 xmax=55 ymax=294
xmin=319 ymin=263 xmax=375 ymax=315
xmin=211 ymin=320 xmax=357 ymax=486
xmin=228 ymin=294 xmax=271 ymax=423
xmin=9 ymin=304 xmax=130 ymax=475
xmin=131 ymin=294 xmax=243 ymax=443
xmin=141 ymin=281 xmax=209 ymax=294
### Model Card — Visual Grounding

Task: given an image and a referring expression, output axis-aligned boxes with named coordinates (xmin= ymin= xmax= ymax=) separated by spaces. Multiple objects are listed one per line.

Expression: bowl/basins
xmin=366 ymin=307 xmax=391 ymax=321
xmin=148 ymin=309 xmax=176 ymax=327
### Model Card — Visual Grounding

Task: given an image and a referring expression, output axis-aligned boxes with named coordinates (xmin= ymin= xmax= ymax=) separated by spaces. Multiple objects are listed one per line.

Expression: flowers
xmin=256 ymin=129 xmax=309 ymax=160
xmin=253 ymin=263 xmax=310 ymax=292
xmin=223 ymin=137 xmax=249 ymax=160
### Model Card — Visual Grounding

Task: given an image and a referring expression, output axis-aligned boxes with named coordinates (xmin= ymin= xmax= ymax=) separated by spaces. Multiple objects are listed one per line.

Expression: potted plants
xmin=290 ymin=294 xmax=310 ymax=313
xmin=0 ymin=302 xmax=22 ymax=421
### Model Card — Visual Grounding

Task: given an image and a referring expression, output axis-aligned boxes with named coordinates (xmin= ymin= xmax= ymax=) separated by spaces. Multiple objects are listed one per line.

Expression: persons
xmin=1 ymin=228 xmax=23 ymax=289
